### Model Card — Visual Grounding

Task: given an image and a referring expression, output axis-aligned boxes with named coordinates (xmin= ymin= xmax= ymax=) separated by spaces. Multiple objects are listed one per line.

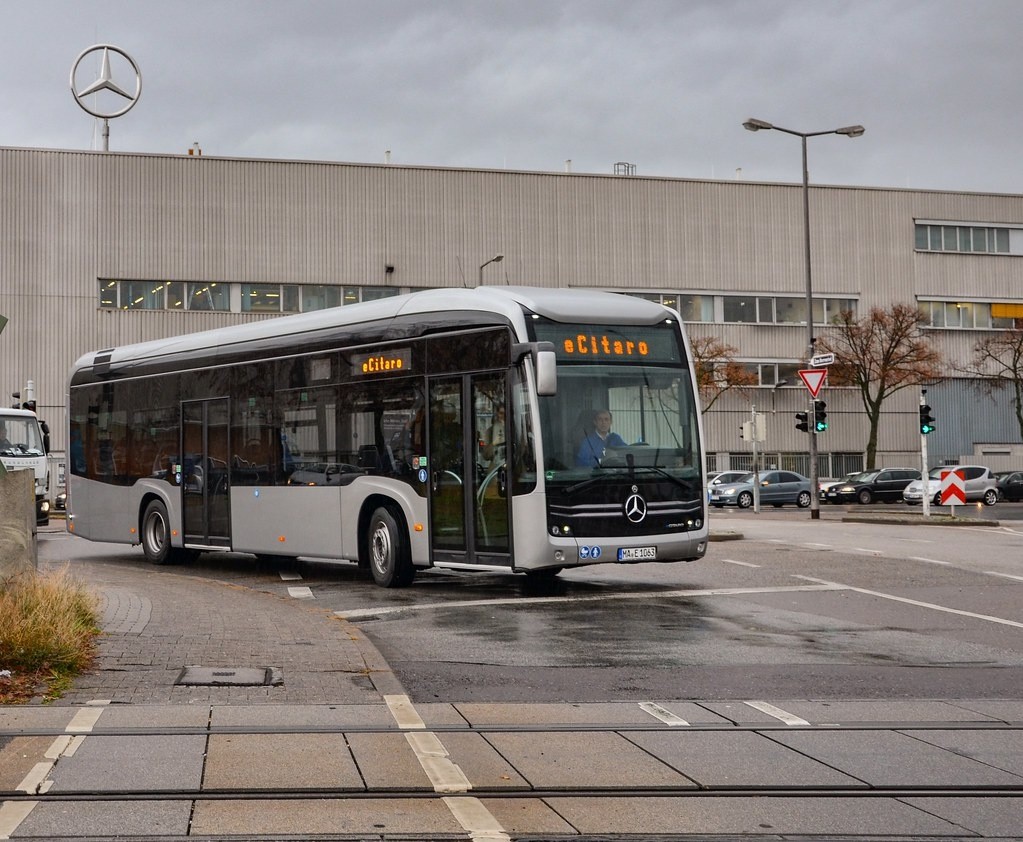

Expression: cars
xmin=710 ymin=470 xmax=820 ymax=509
xmin=705 ymin=471 xmax=753 ymax=489
xmin=902 ymin=464 xmax=999 ymax=507
xmin=992 ymin=471 xmax=1023 ymax=502
xmin=818 ymin=472 xmax=864 ymax=501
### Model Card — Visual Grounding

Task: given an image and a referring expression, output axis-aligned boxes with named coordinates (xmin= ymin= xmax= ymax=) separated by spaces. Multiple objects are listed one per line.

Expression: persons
xmin=0 ymin=427 xmax=16 ymax=451
xmin=576 ymin=410 xmax=629 ymax=468
xmin=434 ymin=397 xmax=529 ymax=488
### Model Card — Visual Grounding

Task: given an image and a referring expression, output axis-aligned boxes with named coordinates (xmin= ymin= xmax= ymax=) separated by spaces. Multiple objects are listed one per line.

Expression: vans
xmin=825 ymin=468 xmax=922 ymax=504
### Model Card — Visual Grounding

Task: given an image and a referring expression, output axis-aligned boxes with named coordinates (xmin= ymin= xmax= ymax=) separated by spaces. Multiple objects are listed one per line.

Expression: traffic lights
xmin=813 ymin=399 xmax=828 ymax=434
xmin=795 ymin=410 xmax=810 ymax=432
xmin=23 ymin=400 xmax=36 ymax=413
xmin=12 ymin=392 xmax=21 ymax=409
xmin=919 ymin=405 xmax=936 ymax=434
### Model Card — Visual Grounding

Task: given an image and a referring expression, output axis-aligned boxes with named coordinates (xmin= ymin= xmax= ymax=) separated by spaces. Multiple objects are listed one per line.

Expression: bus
xmin=65 ymin=285 xmax=711 ymax=588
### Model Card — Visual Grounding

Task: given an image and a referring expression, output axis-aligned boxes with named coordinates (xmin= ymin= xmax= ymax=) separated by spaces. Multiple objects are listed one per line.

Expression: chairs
xmin=356 ymin=444 xmax=387 ymax=472
xmin=77 ymin=422 xmax=274 ymax=497
xmin=567 ymin=407 xmax=601 ymax=462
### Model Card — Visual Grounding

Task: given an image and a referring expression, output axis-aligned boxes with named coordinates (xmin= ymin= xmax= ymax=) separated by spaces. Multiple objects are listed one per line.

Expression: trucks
xmin=0 ymin=408 xmax=53 ymax=526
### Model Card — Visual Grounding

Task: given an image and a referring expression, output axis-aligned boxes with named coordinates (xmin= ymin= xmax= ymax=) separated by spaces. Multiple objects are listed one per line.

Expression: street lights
xmin=772 ymin=380 xmax=789 ymax=470
xmin=743 ymin=119 xmax=865 ymax=519
xmin=478 ymin=255 xmax=504 ymax=286
